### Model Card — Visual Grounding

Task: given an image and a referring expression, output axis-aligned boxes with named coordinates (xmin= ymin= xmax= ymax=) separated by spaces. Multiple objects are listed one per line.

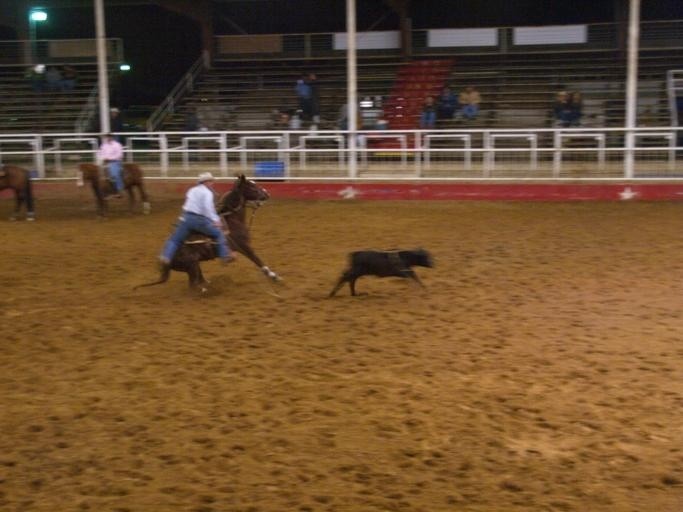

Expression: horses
xmin=0 ymin=166 xmax=36 ymax=222
xmin=132 ymin=172 xmax=285 ymax=296
xmin=73 ymin=163 xmax=152 ymax=222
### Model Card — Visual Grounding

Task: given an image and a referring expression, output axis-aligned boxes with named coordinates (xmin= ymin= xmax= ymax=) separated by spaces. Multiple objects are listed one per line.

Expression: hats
xmin=198 ymin=172 xmax=214 ymax=183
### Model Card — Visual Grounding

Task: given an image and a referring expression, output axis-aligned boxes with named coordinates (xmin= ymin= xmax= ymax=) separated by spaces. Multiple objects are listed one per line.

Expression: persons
xmin=570 ymin=91 xmax=583 ymax=125
xmin=438 ymin=87 xmax=457 ymax=119
xmin=553 ymin=91 xmax=569 ymax=120
xmin=25 ymin=60 xmax=78 ymax=93
xmin=419 ymin=95 xmax=436 ymax=128
xmin=95 ymin=132 xmax=124 ymax=200
xmin=108 ymin=108 xmax=124 ymax=146
xmin=272 ymin=74 xmax=364 ymax=140
xmin=157 ymin=171 xmax=238 ymax=267
xmin=458 ymin=84 xmax=482 ymax=119
xmin=182 ymin=106 xmax=207 ymax=132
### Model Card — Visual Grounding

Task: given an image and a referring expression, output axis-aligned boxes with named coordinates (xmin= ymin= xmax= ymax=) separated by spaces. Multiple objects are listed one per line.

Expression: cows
xmin=328 ymin=246 xmax=435 ymax=298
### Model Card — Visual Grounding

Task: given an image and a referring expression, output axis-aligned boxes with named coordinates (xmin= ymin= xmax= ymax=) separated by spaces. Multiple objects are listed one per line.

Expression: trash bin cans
xmin=252 ymin=159 xmax=284 ymax=177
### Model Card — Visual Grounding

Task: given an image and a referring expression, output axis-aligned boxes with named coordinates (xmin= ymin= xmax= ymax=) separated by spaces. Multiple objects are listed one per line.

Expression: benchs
xmin=0 ymin=68 xmax=97 ymax=133
xmin=426 ymin=53 xmax=683 ymax=128
xmin=159 ymin=61 xmax=408 ymax=131
